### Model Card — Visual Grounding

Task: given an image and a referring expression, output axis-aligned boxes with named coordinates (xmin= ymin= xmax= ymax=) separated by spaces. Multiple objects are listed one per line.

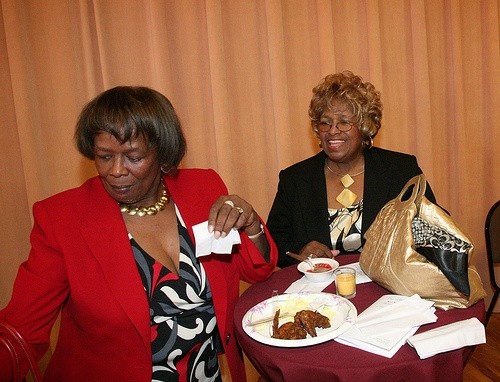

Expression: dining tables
xmin=234 ymin=254 xmax=486 ymax=382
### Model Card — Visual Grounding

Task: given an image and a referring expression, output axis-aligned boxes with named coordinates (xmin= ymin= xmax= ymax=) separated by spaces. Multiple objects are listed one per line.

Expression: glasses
xmin=316 ymin=120 xmax=359 ymax=133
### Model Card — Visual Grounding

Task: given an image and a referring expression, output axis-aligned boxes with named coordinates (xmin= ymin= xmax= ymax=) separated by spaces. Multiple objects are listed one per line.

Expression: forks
xmin=286 ymin=252 xmax=331 ymax=272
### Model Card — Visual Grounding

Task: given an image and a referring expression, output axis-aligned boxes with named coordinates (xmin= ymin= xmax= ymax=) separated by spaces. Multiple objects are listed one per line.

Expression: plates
xmin=242 ymin=292 xmax=357 ymax=347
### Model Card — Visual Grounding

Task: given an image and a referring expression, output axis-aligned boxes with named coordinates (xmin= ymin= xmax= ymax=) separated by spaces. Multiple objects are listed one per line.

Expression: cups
xmin=334 ymin=267 xmax=356 ymax=298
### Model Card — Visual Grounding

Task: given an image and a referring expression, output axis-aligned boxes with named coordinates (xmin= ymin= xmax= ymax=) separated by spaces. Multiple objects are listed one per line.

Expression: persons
xmin=0 ymin=86 xmax=278 ymax=382
xmin=266 ymin=71 xmax=451 ymax=266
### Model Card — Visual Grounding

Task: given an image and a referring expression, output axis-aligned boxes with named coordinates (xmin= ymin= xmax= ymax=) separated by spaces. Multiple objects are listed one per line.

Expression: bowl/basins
xmin=297 ymin=258 xmax=339 ymax=282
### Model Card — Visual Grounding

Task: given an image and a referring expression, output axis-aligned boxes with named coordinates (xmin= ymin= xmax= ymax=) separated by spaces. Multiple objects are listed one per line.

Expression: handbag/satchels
xmin=358 ymin=172 xmax=487 ymax=311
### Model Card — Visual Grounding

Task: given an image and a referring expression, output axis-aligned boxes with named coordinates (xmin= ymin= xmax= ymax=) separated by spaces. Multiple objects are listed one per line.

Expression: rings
xmin=236 ymin=207 xmax=243 ymax=213
xmin=309 ymin=254 xmax=313 ymax=257
xmin=225 ymin=201 xmax=234 ymax=208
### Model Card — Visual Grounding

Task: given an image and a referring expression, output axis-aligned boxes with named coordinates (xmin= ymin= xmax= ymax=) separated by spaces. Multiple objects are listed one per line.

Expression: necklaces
xmin=325 ymin=161 xmax=365 ymax=208
xmin=121 ymin=179 xmax=169 ymax=216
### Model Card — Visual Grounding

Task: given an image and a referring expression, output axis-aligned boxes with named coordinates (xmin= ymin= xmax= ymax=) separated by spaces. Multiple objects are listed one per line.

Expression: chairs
xmin=462 ymin=201 xmax=500 ymax=368
